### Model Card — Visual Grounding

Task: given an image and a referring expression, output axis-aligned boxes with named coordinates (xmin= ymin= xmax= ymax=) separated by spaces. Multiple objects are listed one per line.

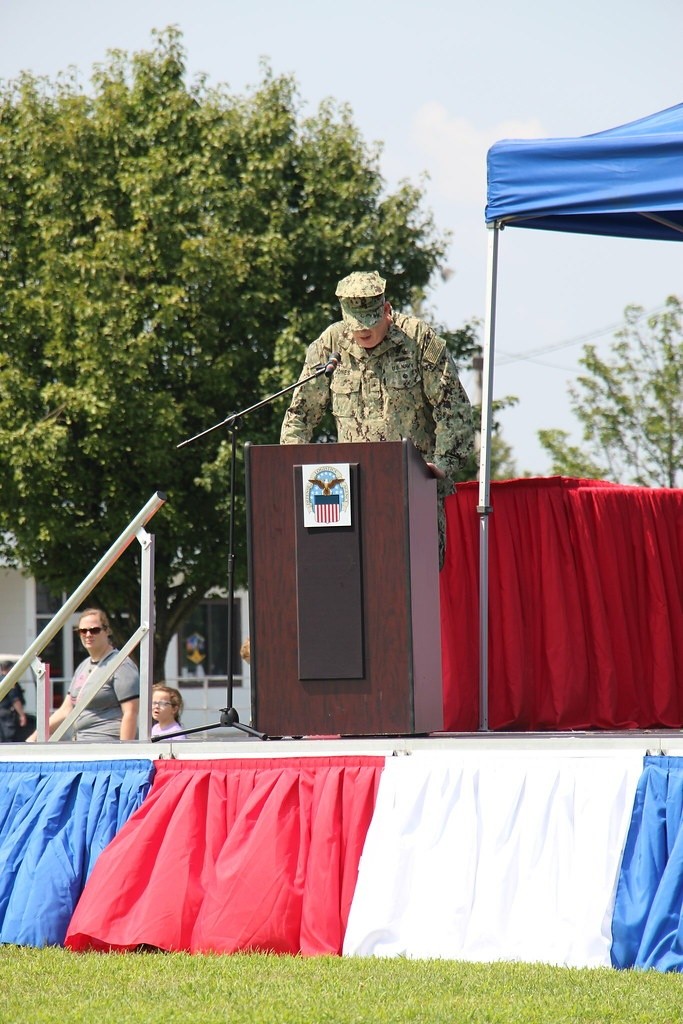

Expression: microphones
xmin=325 ymin=352 xmax=341 ymax=377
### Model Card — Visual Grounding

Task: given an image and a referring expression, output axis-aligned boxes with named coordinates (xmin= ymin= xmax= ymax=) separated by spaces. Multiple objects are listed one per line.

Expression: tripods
xmin=152 ymin=365 xmax=330 ymax=741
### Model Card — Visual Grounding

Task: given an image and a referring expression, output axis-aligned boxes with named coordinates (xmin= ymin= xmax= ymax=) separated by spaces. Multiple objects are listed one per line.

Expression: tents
xmin=471 ymin=100 xmax=683 ymax=732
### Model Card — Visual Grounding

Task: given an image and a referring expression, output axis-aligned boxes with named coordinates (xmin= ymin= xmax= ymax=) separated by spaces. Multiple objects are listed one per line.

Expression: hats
xmin=334 ymin=272 xmax=388 ymax=331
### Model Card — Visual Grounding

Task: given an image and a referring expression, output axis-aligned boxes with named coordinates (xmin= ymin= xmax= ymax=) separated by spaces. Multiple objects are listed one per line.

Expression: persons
xmin=0 ymin=606 xmax=190 ymax=743
xmin=278 ymin=269 xmax=475 ymax=573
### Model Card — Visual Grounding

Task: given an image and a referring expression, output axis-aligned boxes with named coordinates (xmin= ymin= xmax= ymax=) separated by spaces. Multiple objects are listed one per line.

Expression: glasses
xmin=152 ymin=701 xmax=174 ymax=708
xmin=78 ymin=627 xmax=105 ymax=635
